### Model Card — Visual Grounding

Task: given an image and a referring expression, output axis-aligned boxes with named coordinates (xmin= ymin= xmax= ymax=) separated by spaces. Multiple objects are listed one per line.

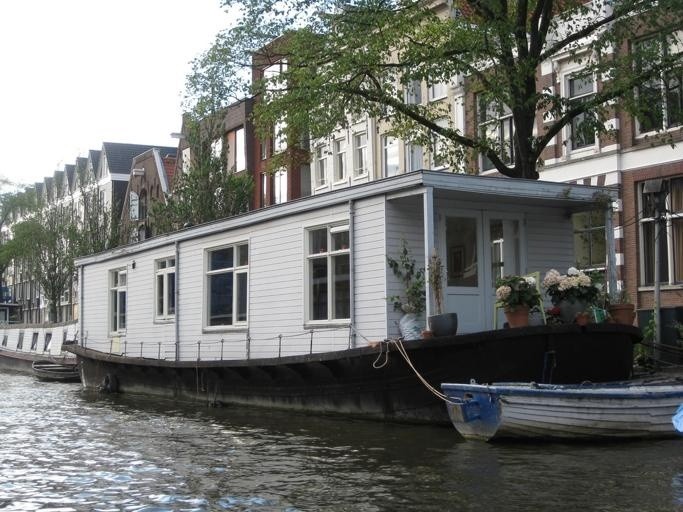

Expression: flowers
xmin=492 ymin=270 xmax=542 ymax=308
xmin=542 ymin=265 xmax=603 ymax=307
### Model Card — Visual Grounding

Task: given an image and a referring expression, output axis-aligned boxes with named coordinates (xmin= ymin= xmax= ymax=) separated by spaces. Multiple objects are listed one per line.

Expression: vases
xmin=503 ymin=302 xmax=533 ymax=327
xmin=428 ymin=312 xmax=458 ymax=337
xmin=558 ymin=298 xmax=586 ymax=321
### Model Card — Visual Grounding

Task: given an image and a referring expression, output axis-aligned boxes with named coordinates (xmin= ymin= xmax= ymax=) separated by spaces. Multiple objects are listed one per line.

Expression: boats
xmin=30 ymin=358 xmax=79 ymax=382
xmin=439 ymin=379 xmax=683 ymax=444
xmin=59 ymin=169 xmax=644 ymax=430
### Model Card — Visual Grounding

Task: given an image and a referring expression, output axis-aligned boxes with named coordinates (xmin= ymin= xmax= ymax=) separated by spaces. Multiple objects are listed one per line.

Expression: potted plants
xmin=609 ymin=282 xmax=636 ymax=326
